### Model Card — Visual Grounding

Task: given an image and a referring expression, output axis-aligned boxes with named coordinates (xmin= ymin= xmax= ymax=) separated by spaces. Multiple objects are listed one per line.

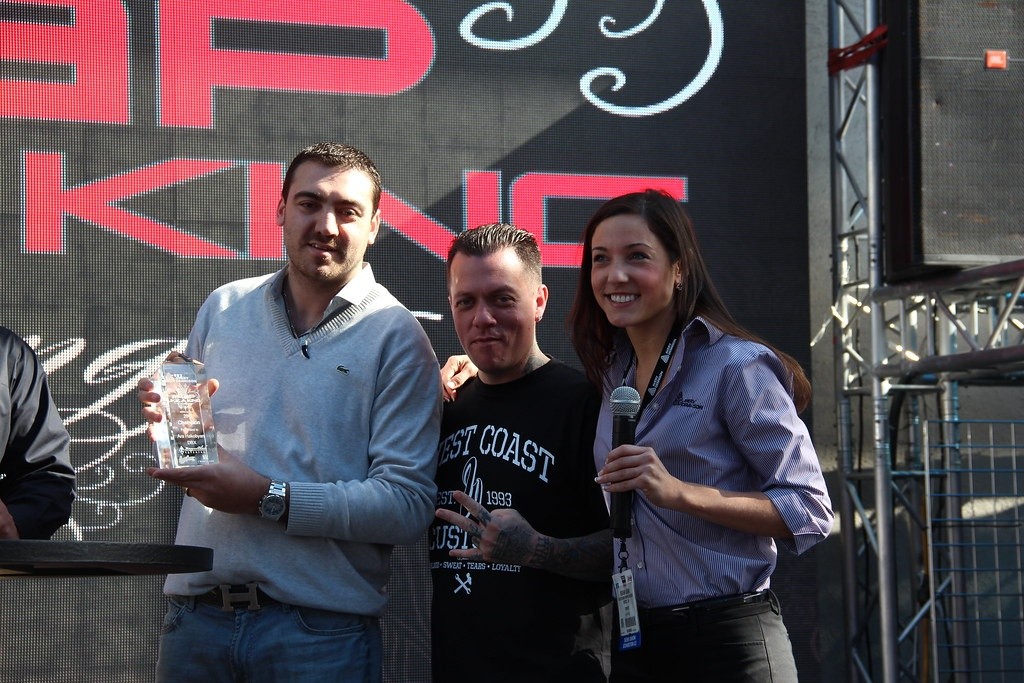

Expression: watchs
xmin=260 ymin=478 xmax=289 ymax=522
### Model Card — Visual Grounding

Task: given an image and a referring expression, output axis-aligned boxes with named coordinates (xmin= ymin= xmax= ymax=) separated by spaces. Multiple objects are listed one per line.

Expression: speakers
xmin=874 ymin=1 xmax=1023 ymax=283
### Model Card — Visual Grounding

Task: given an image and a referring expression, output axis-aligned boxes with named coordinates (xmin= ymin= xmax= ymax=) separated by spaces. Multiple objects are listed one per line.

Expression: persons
xmin=432 ymin=186 xmax=834 ymax=681
xmin=427 ymin=220 xmax=614 ymax=683
xmin=0 ymin=321 xmax=78 ymax=541
xmin=136 ymin=140 xmax=446 ymax=682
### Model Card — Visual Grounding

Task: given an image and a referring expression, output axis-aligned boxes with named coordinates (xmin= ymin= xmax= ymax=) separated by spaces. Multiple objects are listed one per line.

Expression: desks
xmin=1 ymin=540 xmax=213 ymax=577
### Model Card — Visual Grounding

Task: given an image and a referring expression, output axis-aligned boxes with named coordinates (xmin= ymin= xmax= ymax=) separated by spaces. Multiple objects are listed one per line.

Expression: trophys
xmin=146 ymin=349 xmax=221 ymax=469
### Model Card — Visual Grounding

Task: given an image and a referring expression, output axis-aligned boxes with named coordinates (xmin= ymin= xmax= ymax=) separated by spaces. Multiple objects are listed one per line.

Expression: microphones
xmin=609 ymin=386 xmax=641 ymax=539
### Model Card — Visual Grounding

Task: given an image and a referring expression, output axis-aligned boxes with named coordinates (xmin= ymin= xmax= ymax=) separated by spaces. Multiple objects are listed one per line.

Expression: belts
xmin=201 ymin=582 xmax=272 ymax=613
xmin=652 ymin=591 xmax=771 ymax=618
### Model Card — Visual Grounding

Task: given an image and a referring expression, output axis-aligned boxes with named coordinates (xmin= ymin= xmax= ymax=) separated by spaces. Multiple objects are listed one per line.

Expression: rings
xmin=184 ymin=487 xmax=192 ymax=498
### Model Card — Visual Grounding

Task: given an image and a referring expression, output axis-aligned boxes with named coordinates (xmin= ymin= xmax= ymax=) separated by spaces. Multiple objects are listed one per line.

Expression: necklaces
xmin=282 ymin=274 xmax=328 ymax=361
